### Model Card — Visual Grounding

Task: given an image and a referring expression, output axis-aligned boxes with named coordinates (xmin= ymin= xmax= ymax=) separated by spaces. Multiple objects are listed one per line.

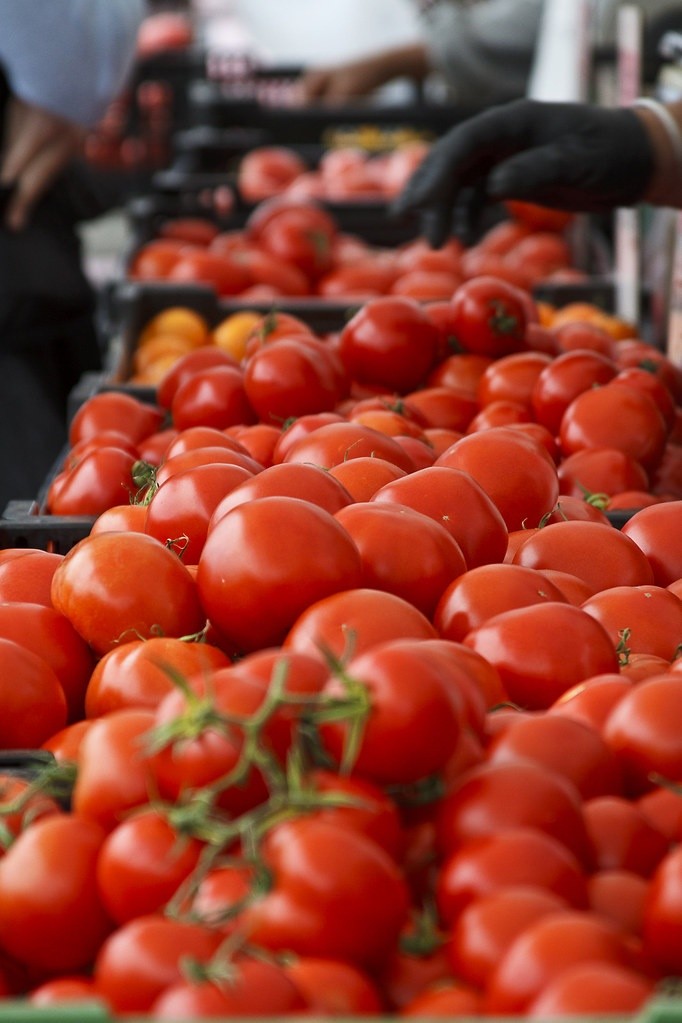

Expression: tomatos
xmin=0 ymin=128 xmax=682 ymax=1016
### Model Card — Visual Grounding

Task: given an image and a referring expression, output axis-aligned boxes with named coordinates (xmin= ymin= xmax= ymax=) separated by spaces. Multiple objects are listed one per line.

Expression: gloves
xmin=385 ymin=99 xmax=680 ymax=250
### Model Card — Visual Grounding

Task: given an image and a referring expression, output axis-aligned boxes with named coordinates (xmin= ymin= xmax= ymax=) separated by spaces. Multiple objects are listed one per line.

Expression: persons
xmin=372 ymin=99 xmax=682 ymax=252
xmin=2 ymin=1 xmax=144 ymax=438
xmin=308 ymin=1 xmax=682 ymax=108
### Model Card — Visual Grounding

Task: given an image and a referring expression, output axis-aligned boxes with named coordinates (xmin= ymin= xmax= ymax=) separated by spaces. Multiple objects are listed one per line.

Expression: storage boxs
xmin=2 ymin=59 xmax=681 ymax=1023
